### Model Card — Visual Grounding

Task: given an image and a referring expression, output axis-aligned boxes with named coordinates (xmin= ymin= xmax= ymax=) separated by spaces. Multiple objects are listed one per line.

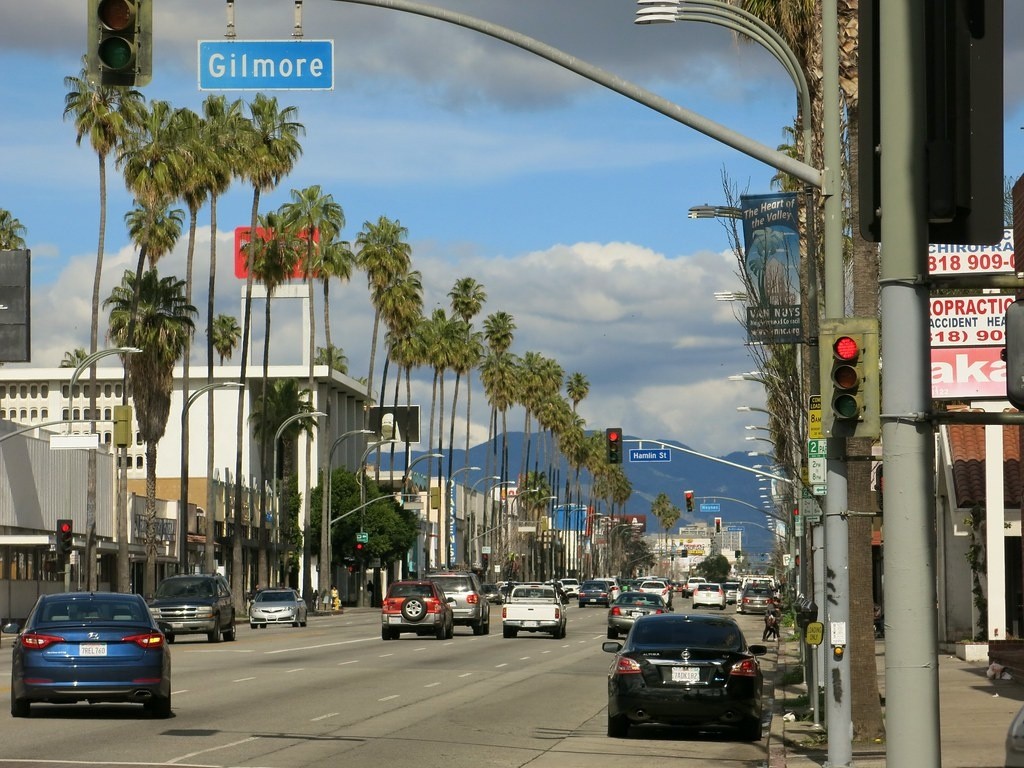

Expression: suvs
xmin=593 ymin=577 xmax=621 ymax=602
xmin=381 ymin=578 xmax=454 ymax=641
xmin=147 ymin=573 xmax=236 ymax=644
xmin=560 ymin=578 xmax=580 ymax=599
xmin=424 ymin=569 xmax=490 ymax=636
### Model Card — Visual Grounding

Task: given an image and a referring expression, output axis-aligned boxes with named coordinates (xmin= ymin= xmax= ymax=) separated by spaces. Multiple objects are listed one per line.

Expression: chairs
xmin=48 ymin=607 xmax=69 ymax=621
xmin=113 ymin=609 xmax=133 ymax=621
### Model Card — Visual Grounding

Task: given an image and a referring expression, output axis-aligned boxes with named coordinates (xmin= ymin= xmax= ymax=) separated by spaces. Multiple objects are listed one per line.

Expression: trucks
xmin=736 ymin=573 xmax=775 ymax=614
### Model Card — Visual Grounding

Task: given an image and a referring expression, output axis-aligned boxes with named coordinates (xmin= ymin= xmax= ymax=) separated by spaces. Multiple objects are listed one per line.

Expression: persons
xmin=626 ymin=597 xmax=632 ymax=603
xmin=332 ymin=586 xmax=339 ymax=609
xmin=762 ymin=597 xmax=783 ymax=642
xmin=252 ymin=585 xmax=262 ymax=598
xmin=367 ymin=580 xmax=374 ymax=607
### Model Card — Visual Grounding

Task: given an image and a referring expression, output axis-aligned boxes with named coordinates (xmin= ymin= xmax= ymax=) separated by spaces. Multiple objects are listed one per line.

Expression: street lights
xmin=482 ymin=481 xmax=517 ymax=572
xmin=528 ymin=495 xmax=643 ymax=579
xmin=712 ymin=290 xmax=822 ymax=730
xmin=327 ymin=429 xmax=377 ymax=590
xmin=444 ymin=466 xmax=485 ymax=572
xmin=467 ymin=475 xmax=501 ymax=573
xmin=359 ymin=439 xmax=401 ymax=607
xmin=401 ymin=452 xmax=446 ymax=505
xmin=68 ymin=346 xmax=144 ymax=434
xmin=180 ymin=381 xmax=244 ymax=577
xmin=273 ymin=411 xmax=328 ymax=589
xmin=632 ymin=0 xmax=826 ymax=732
xmin=509 ymin=489 xmax=538 ymax=552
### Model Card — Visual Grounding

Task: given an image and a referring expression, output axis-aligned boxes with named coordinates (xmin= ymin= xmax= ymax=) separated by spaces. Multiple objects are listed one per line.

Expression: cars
xmin=481 ymin=583 xmax=503 ymax=605
xmin=606 ymin=591 xmax=675 ymax=639
xmin=602 ymin=612 xmax=767 ymax=741
xmin=578 ymin=581 xmax=612 ymax=608
xmin=617 ymin=576 xmax=687 ymax=598
xmin=249 ymin=588 xmax=307 ymax=629
xmin=640 ymin=580 xmax=669 ymax=606
xmin=742 ymin=583 xmax=775 ymax=615
xmin=692 ymin=583 xmax=726 ymax=611
xmin=495 ymin=580 xmax=565 ymax=604
xmin=2 ymin=590 xmax=172 ymax=717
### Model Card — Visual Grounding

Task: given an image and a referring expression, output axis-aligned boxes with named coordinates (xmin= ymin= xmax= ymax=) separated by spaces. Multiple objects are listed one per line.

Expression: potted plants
xmin=955 ymin=505 xmax=1012 ymax=663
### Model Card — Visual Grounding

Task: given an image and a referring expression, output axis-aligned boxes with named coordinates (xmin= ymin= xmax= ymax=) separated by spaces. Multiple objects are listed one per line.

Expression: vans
xmin=722 ymin=582 xmax=739 ymax=603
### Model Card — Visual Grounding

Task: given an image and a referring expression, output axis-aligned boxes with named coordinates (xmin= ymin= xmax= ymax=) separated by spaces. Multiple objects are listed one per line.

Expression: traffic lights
xmin=482 ymin=554 xmax=488 ymax=568
xmin=44 ymin=560 xmax=56 ymax=572
xmin=56 ymin=519 xmax=73 ymax=556
xmin=357 ymin=543 xmax=363 ymax=550
xmin=87 ymin=1 xmax=154 ymax=86
xmin=819 ymin=317 xmax=880 ymax=439
xmin=684 ymin=490 xmax=695 ymax=512
xmin=714 ymin=517 xmax=722 ymax=535
xmin=605 ymin=427 xmax=623 ymax=465
xmin=794 ymin=504 xmax=798 ymax=515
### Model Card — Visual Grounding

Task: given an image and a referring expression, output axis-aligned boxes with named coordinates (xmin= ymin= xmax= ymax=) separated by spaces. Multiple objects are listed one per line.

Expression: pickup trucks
xmin=501 ymin=583 xmax=566 ymax=639
xmin=685 ymin=577 xmax=706 ymax=599
